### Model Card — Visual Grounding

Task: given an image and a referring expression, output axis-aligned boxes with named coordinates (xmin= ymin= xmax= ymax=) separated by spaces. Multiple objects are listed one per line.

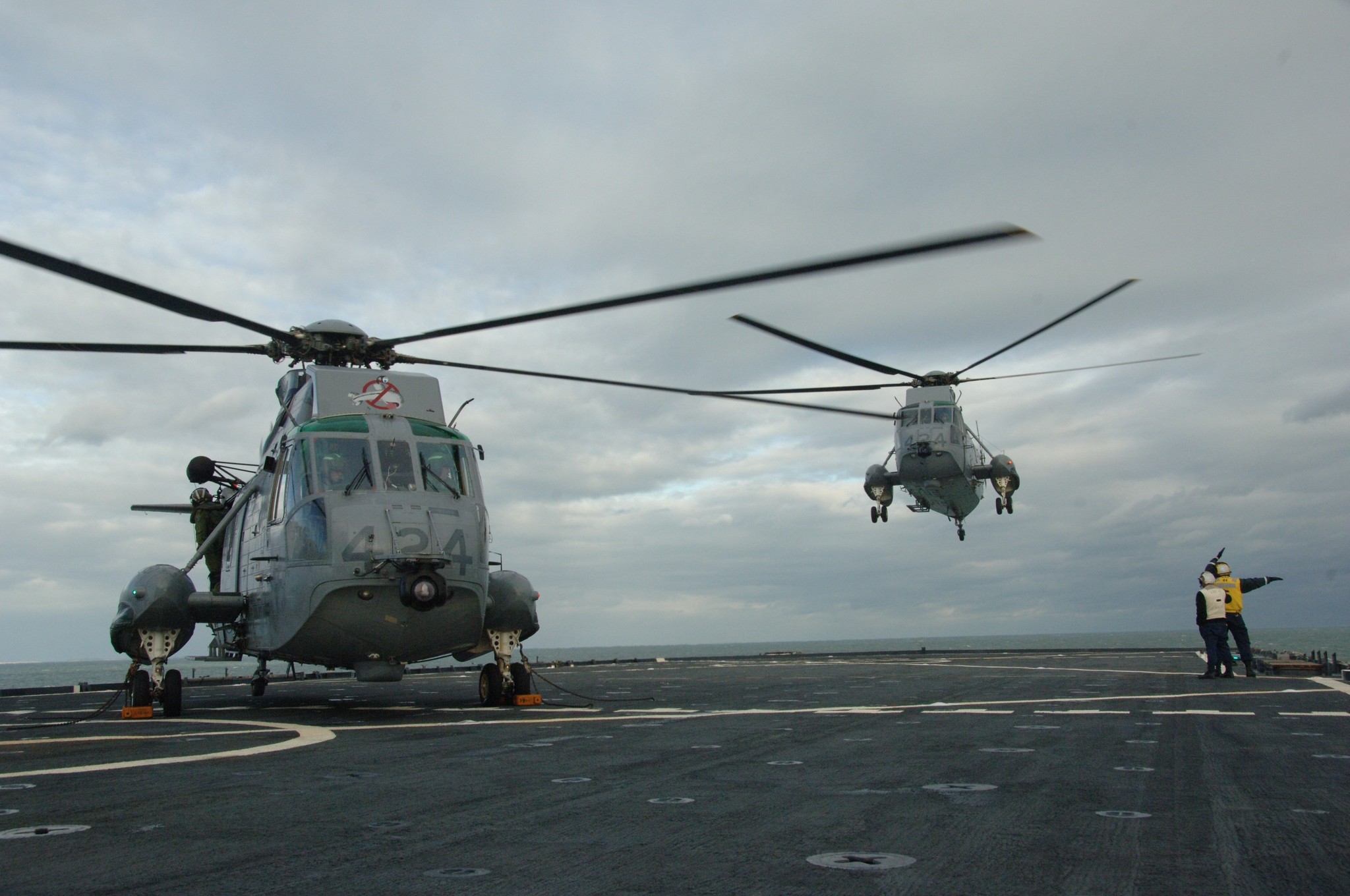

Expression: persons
xmin=190 ymin=487 xmax=228 ymax=594
xmin=310 ymin=438 xmax=456 ymax=498
xmin=1195 ymin=571 xmax=1234 ymax=678
xmin=1205 ymin=547 xmax=1283 ymax=677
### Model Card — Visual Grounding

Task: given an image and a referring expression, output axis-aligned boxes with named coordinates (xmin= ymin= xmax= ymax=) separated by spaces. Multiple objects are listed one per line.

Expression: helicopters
xmin=686 ymin=274 xmax=1203 ymax=541
xmin=0 ymin=225 xmax=1039 ymax=728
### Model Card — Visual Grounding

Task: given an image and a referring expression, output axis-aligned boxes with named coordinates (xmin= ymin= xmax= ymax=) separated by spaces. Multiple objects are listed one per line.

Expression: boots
xmin=1220 ymin=665 xmax=1235 ymax=679
xmin=1197 ymin=663 xmax=1216 ymax=679
xmin=1215 ymin=659 xmax=1223 ymax=677
xmin=1242 ymin=660 xmax=1256 ymax=677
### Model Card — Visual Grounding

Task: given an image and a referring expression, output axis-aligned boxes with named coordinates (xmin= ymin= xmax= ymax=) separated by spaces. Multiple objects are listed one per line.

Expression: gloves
xmin=1269 ymin=577 xmax=1283 ymax=582
xmin=1216 ymin=547 xmax=1225 ymax=559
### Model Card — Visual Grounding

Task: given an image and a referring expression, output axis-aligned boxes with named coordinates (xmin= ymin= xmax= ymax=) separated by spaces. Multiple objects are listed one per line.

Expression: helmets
xmin=1215 ymin=562 xmax=1232 ymax=576
xmin=322 ymin=452 xmax=348 ymax=471
xmin=190 ymin=487 xmax=213 ymax=508
xmin=1198 ymin=571 xmax=1215 ymax=589
xmin=425 ymin=452 xmax=453 ymax=470
